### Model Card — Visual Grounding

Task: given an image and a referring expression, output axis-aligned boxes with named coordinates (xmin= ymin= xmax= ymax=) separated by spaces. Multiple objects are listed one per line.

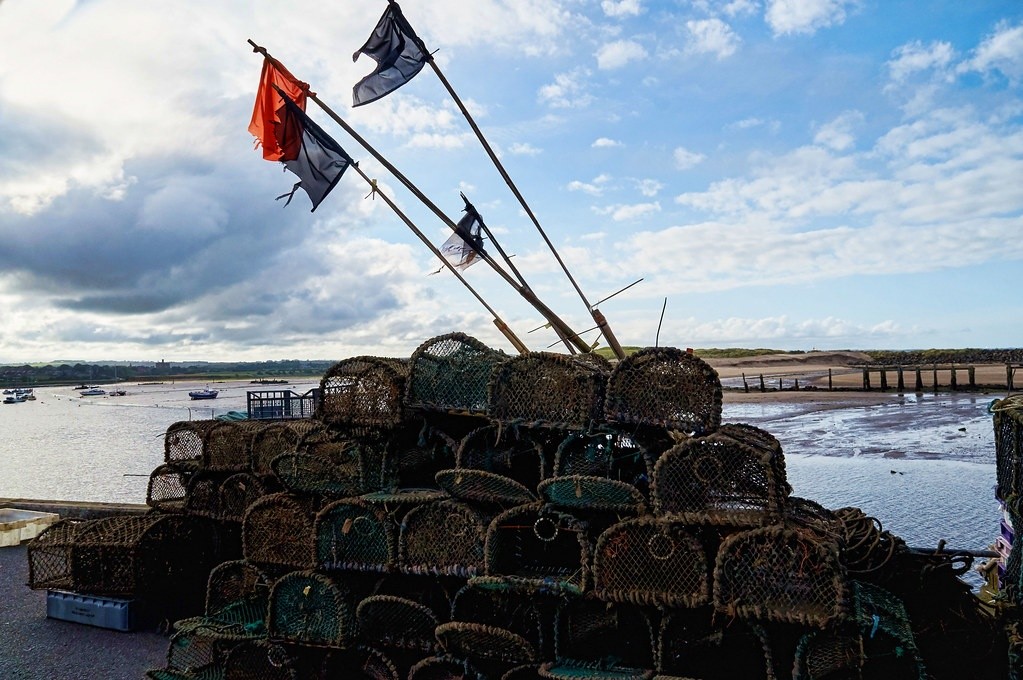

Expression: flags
xmin=248 ymin=55 xmax=350 ymax=213
xmin=439 ymin=205 xmax=487 ymax=272
xmin=352 ymin=3 xmax=434 ymax=107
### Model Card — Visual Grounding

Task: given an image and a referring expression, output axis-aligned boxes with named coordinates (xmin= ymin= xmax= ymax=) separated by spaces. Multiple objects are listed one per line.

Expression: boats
xmin=79 ymin=388 xmax=106 ymax=395
xmin=250 ymin=376 xmax=288 ymax=384
xmin=189 ymin=389 xmax=219 ymax=400
xmin=2 ymin=387 xmax=37 ymax=403
xmin=75 ymin=380 xmax=100 ymax=390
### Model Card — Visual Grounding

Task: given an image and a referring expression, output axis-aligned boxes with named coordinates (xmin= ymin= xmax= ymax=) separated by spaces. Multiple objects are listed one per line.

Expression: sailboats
xmin=109 ymin=364 xmax=126 ymax=396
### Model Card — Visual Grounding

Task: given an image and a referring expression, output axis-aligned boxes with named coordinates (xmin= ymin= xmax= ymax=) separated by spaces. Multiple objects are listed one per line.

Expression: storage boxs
xmin=47 ymin=588 xmax=139 ymax=633
xmin=0 ymin=507 xmax=60 ymax=548
